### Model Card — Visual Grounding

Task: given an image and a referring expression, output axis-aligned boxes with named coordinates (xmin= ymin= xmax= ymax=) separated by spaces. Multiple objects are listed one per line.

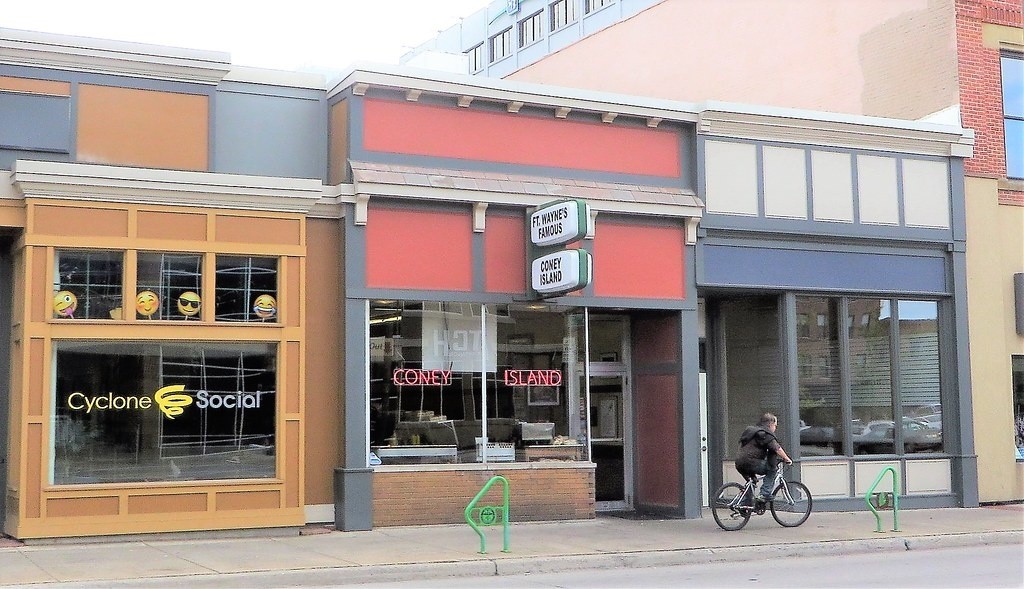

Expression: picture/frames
xmin=506 ymin=333 xmax=534 ymax=370
xmin=598 ymin=395 xmax=619 ymax=437
xmin=599 ymin=352 xmax=618 ymax=379
xmin=527 ymin=375 xmax=559 ymax=406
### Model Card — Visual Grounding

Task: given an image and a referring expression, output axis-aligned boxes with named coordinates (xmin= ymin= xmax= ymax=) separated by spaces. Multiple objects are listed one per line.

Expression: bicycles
xmin=710 ymin=458 xmax=814 ymax=533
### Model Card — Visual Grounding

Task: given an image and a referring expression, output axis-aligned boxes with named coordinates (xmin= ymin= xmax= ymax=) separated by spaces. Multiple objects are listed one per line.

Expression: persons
xmin=735 ymin=413 xmax=792 ymax=517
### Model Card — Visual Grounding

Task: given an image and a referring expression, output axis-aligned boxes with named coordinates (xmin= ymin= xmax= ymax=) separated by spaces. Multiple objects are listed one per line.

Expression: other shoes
xmin=756 ymin=493 xmax=775 ymax=502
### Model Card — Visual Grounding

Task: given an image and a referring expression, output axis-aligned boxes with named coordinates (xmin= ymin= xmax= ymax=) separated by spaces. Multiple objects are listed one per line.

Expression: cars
xmin=797 ymin=404 xmax=946 ymax=455
